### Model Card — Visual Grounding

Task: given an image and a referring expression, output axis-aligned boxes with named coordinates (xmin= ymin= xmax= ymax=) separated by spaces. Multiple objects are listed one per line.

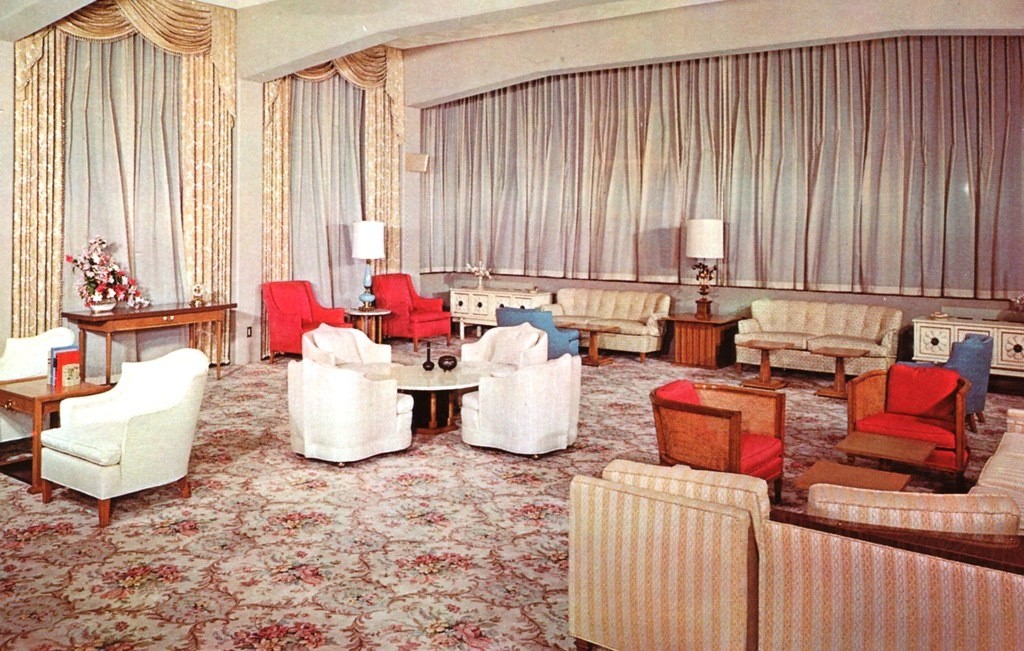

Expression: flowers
xmin=66 ymin=235 xmax=153 ymax=310
xmin=1011 ymin=293 xmax=1024 ymax=310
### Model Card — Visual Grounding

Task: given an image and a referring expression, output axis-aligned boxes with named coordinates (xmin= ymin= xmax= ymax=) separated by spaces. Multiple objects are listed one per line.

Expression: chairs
xmin=496 ymin=307 xmax=580 ymax=361
xmin=848 ymin=365 xmax=972 ymax=494
xmin=371 ymin=273 xmax=451 ymax=352
xmin=39 ymin=348 xmax=209 ymax=527
xmin=649 ymin=380 xmax=786 ymax=504
xmin=0 ymin=326 xmax=75 ymax=449
xmin=897 ymin=333 xmax=994 ymax=432
xmin=263 ymin=281 xmax=353 ymax=364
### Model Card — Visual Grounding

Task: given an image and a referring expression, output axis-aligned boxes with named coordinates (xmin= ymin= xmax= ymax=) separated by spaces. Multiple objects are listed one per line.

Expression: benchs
xmin=734 ymin=298 xmax=903 ymax=376
xmin=540 ymin=288 xmax=671 ymax=362
xmin=567 ymin=409 xmax=1024 ymax=651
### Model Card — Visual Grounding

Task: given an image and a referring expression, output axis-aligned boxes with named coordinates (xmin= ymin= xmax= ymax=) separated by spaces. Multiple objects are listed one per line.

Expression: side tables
xmin=660 ymin=313 xmax=743 ymax=370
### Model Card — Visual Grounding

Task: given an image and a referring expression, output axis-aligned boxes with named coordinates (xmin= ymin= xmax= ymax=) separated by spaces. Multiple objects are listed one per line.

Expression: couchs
xmin=460 ymin=322 xmax=548 ymax=379
xmin=302 ymin=323 xmax=405 ymax=378
xmin=461 ymin=354 xmax=582 ymax=459
xmin=287 ymin=358 xmax=415 ymax=468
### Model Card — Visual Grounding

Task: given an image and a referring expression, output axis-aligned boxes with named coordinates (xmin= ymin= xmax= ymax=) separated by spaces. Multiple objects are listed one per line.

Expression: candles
xmin=479 ymin=239 xmax=483 ymax=261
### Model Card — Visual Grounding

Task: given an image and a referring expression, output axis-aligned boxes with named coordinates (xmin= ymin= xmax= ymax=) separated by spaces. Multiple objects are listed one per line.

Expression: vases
xmin=423 ymin=341 xmax=435 ymax=371
xmin=90 ymin=304 xmax=116 ymax=313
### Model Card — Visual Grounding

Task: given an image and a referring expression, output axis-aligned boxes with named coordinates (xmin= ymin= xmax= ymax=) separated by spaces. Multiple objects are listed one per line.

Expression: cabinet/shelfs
xmin=449 ymin=287 xmax=552 ymax=340
xmin=912 ymin=315 xmax=1024 ymax=378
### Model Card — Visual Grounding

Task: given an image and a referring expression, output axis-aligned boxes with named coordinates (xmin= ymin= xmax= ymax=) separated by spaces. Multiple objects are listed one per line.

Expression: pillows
xmin=271 ymin=282 xmax=312 ymax=324
xmin=379 ymin=278 xmax=413 ymax=311
xmin=492 ymin=328 xmax=539 ymax=362
xmin=312 ymin=331 xmax=361 ymax=365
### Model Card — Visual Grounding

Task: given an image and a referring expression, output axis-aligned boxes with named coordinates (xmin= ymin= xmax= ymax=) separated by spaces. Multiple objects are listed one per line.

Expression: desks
xmin=365 ymin=365 xmax=482 ymax=435
xmin=0 ymin=375 xmax=111 ymax=494
xmin=736 ymin=341 xmax=796 ymax=390
xmin=556 ymin=322 xmax=575 ymax=328
xmin=346 ymin=308 xmax=391 ymax=344
xmin=569 ymin=323 xmax=622 ymax=367
xmin=834 ymin=432 xmax=937 ymax=473
xmin=62 ymin=302 xmax=237 ymax=385
xmin=811 ymin=346 xmax=871 ymax=400
xmin=793 ymin=459 xmax=912 ymax=501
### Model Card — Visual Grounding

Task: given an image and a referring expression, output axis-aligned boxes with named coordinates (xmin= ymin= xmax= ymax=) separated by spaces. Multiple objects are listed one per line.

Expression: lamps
xmin=352 ymin=221 xmax=385 ymax=312
xmin=685 ymin=219 xmax=725 ymax=321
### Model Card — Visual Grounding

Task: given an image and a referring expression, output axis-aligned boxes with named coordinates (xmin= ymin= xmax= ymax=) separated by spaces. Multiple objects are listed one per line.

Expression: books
xmin=47 ymin=344 xmax=81 ymax=388
